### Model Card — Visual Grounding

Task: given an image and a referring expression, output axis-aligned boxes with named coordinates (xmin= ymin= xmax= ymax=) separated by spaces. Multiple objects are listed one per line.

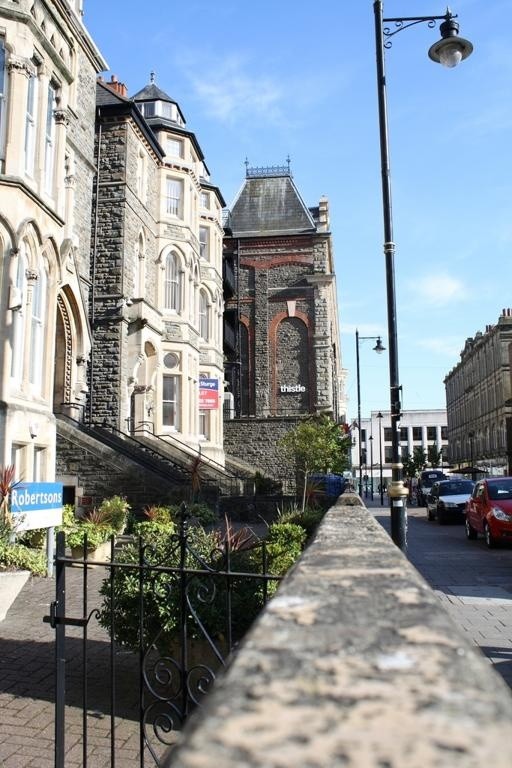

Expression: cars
xmin=466 ymin=476 xmax=512 ymax=548
xmin=426 ymin=478 xmax=474 ymax=524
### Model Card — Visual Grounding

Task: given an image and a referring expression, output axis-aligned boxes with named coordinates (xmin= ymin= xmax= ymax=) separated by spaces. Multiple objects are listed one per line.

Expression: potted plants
xmin=97 ymin=522 xmax=309 ymax=703
xmin=63 ymin=496 xmax=128 ymax=569
xmin=98 ymin=494 xmax=132 ymax=539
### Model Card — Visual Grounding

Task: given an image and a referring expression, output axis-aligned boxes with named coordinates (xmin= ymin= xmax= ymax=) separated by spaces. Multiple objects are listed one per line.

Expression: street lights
xmin=355 ymin=330 xmax=386 ymax=507
xmin=373 ymin=2 xmax=473 ymax=547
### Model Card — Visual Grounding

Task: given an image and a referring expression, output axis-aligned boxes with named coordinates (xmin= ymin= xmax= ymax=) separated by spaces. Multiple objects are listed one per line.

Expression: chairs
xmin=489 ymin=486 xmax=498 ymax=497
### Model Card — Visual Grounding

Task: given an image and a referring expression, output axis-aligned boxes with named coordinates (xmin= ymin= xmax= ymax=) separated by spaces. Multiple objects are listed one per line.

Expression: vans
xmin=417 ymin=470 xmax=447 ymax=506
